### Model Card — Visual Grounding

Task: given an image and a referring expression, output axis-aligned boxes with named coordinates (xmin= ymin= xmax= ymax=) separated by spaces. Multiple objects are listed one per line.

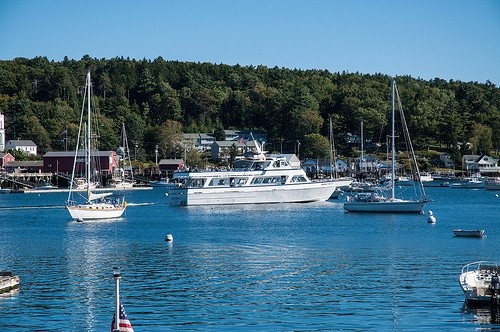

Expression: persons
xmin=227 ymin=164 xmax=230 ymax=171
xmin=214 ymin=166 xmax=218 ymax=171
xmin=190 ymin=165 xmax=198 ymax=172
xmin=209 ymin=166 xmax=213 ymax=171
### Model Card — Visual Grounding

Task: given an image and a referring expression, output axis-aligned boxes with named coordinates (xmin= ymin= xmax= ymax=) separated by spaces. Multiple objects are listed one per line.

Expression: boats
xmin=380 ymin=172 xmax=449 ymax=183
xmin=450 ymin=175 xmax=500 ymax=190
xmin=452 ymin=229 xmax=484 ymax=237
xmin=30 ymin=179 xmax=58 ymax=189
xmin=166 ymin=132 xmax=354 ymax=206
xmin=459 ymin=260 xmax=500 ymax=307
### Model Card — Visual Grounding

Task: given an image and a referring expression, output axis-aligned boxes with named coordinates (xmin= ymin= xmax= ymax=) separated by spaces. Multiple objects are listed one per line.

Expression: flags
xmin=111 ymin=302 xmax=134 ymax=332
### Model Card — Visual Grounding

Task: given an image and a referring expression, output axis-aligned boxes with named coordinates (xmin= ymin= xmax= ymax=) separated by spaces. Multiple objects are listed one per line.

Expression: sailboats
xmin=64 ymin=70 xmax=128 ymax=220
xmin=68 ymin=122 xmax=100 ymax=189
xmin=341 ymin=79 xmax=433 ymax=213
xmin=106 ymin=123 xmax=137 ymax=188
